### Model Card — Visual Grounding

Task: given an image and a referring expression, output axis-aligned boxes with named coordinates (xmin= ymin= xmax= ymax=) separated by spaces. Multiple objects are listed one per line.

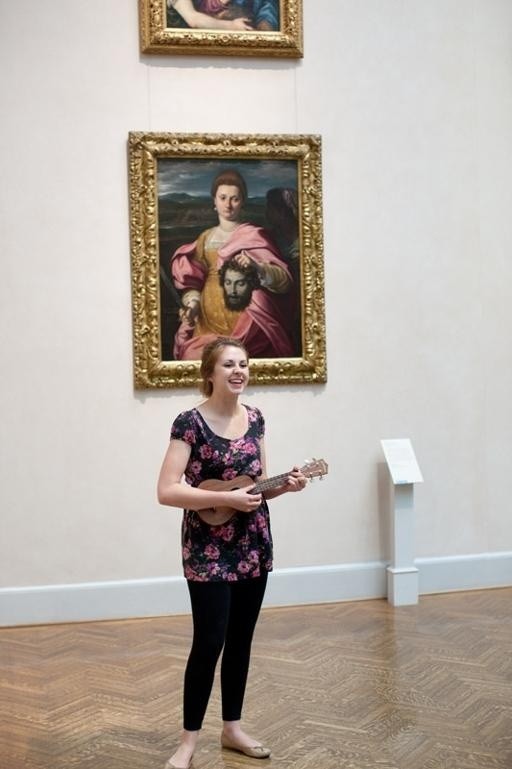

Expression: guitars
xmin=197 ymin=457 xmax=329 ymax=525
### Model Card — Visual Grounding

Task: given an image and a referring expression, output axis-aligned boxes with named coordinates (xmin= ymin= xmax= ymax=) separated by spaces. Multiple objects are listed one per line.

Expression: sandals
xmin=220 ymin=728 xmax=270 ymax=757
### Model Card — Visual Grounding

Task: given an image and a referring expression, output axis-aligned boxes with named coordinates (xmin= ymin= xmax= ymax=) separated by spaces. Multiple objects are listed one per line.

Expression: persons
xmin=168 ymin=0 xmax=255 ymax=32
xmin=170 ymin=167 xmax=298 ymax=360
xmin=219 ymin=256 xmax=262 ymax=314
xmin=154 ymin=332 xmax=311 ymax=767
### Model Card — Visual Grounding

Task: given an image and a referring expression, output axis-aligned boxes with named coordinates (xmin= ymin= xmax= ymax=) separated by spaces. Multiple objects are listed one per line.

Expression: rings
xmin=247 ymin=507 xmax=252 ymax=514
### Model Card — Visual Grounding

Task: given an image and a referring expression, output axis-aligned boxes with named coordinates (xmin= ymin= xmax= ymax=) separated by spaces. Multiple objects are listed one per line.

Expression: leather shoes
xmin=163 ymin=753 xmax=194 ymax=769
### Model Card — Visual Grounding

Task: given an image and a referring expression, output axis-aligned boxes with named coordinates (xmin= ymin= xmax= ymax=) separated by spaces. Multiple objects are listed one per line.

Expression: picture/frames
xmin=126 ymin=131 xmax=327 ymax=389
xmin=137 ymin=0 xmax=304 ymax=60
